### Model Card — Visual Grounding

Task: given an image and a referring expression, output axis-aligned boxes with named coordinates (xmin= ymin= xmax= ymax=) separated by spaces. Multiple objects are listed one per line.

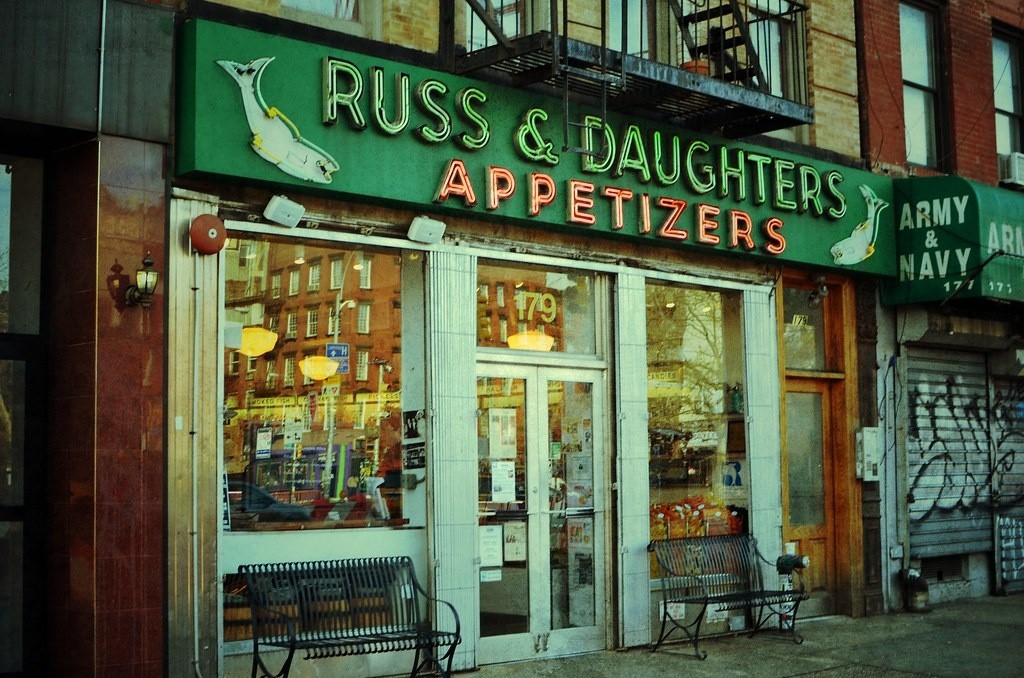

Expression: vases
xmin=679 ymin=59 xmax=708 ymax=76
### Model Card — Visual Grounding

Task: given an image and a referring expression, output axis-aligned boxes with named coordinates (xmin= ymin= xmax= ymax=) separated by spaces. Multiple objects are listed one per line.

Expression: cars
xmin=223 ymin=481 xmax=310 ymax=522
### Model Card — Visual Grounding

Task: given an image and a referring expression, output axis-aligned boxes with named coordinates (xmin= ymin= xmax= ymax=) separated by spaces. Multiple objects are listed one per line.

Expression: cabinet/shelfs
xmin=649 ymin=413 xmax=747 ymax=460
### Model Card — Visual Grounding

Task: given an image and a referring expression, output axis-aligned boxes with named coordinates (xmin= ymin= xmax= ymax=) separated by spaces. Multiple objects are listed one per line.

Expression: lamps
xmin=809 ymin=285 xmax=829 ymax=312
xmin=126 ymin=250 xmax=159 ymax=310
xmin=106 ymin=256 xmax=131 ymax=314
xmin=298 ymin=355 xmax=340 ymax=380
xmin=507 ymin=329 xmax=555 ymax=352
xmin=236 ymin=326 xmax=279 ymax=357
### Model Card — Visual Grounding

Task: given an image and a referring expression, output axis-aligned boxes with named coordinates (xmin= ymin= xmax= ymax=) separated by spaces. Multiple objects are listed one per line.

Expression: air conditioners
xmin=998 ymin=152 xmax=1024 ymax=186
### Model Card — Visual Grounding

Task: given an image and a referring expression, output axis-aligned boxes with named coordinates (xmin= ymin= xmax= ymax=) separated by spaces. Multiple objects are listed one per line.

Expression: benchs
xmin=646 ymin=532 xmax=811 ymax=661
xmin=238 ymin=555 xmax=463 ymax=678
